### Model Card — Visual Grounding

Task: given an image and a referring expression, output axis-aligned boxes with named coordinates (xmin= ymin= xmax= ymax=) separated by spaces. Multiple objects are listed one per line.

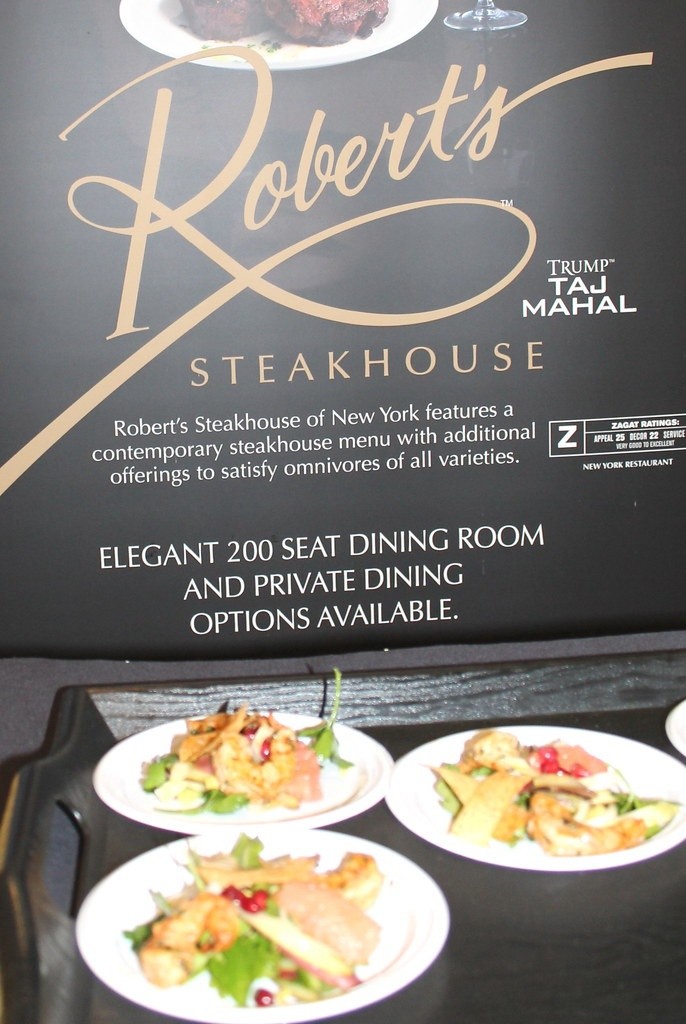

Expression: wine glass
xmin=443 ymin=0 xmax=529 ymax=30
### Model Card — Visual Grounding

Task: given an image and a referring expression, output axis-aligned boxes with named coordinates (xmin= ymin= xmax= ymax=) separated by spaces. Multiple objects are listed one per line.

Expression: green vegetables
xmin=431 ymin=761 xmax=645 ymax=843
xmin=145 ymin=665 xmax=355 ymax=813
xmin=123 ymin=836 xmax=328 ymax=1005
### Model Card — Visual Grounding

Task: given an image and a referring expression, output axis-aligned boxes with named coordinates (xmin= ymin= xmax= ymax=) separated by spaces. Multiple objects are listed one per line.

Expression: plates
xmin=665 ymin=699 xmax=686 ymax=756
xmin=92 ymin=711 xmax=395 ymax=835
xmin=384 ymin=726 xmax=686 ymax=873
xmin=74 ymin=830 xmax=451 ymax=1024
xmin=118 ymin=0 xmax=439 ymax=68
